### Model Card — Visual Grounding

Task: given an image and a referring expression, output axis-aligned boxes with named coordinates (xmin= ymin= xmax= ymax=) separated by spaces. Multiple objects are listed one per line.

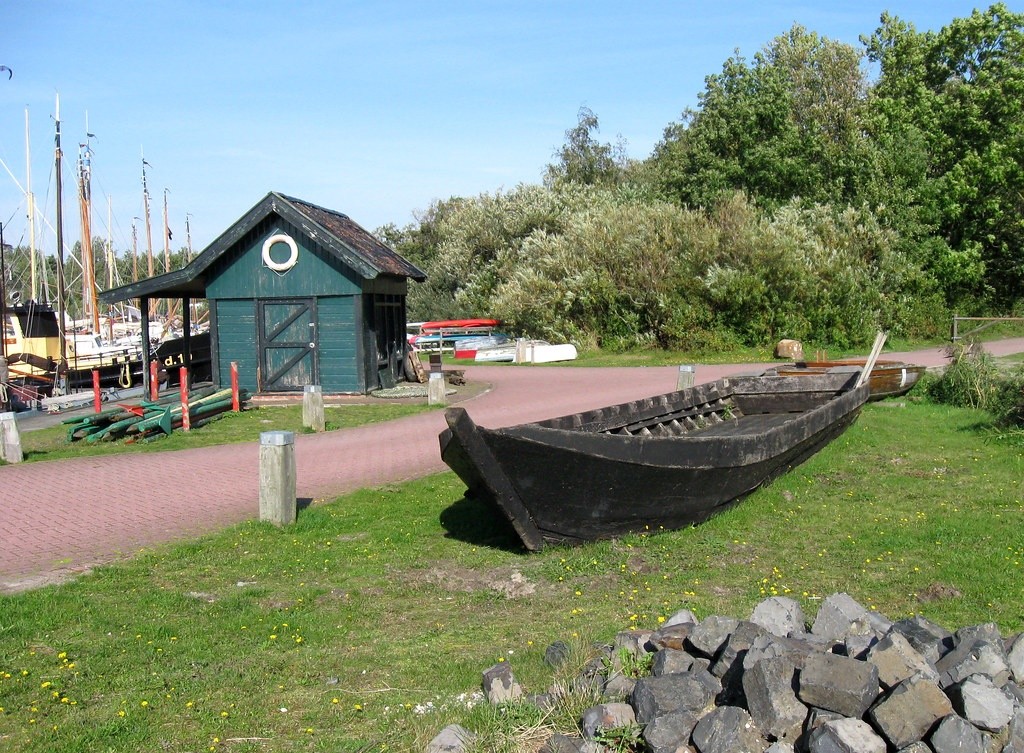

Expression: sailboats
xmin=0 ymin=97 xmax=210 ymax=414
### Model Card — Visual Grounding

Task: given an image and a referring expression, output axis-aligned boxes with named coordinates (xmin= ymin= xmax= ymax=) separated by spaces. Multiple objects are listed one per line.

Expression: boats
xmin=405 ymin=320 xmax=578 ymax=363
xmin=762 ymin=359 xmax=928 ymax=402
xmin=437 ymin=376 xmax=871 ymax=551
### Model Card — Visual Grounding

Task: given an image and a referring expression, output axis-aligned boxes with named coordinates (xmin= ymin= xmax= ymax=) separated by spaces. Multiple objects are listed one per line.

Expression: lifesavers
xmin=260 ymin=233 xmax=298 ymax=271
